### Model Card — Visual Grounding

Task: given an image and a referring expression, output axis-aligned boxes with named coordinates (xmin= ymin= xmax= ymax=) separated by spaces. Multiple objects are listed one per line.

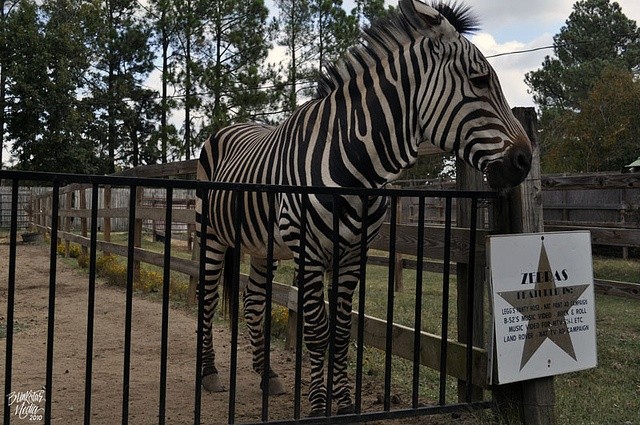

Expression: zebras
xmin=196 ymin=0 xmax=532 ymax=416
